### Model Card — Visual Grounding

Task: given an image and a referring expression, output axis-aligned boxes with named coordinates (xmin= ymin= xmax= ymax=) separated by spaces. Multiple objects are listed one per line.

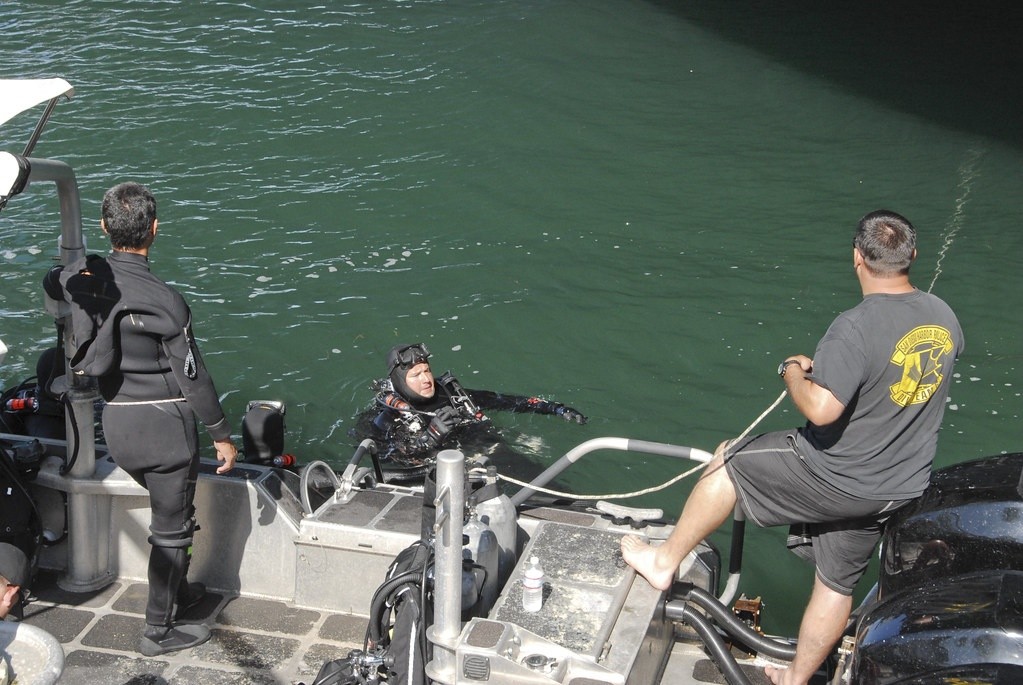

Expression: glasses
xmin=852 ymin=236 xmax=865 ymax=259
xmin=394 ymin=343 xmax=431 ymax=370
xmin=7 ymin=584 xmax=25 ymax=601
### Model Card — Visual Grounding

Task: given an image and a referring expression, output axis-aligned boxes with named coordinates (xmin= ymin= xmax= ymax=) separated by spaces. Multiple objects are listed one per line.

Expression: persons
xmin=17 ymin=346 xmax=66 ymax=440
xmin=371 ymin=341 xmax=584 ymax=454
xmin=0 ymin=542 xmax=30 ymax=619
xmin=43 ymin=181 xmax=235 ymax=656
xmin=621 ymin=209 xmax=965 ymax=685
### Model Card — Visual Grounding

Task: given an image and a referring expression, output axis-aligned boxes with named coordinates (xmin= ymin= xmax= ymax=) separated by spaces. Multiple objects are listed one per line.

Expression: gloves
xmin=556 ymin=406 xmax=586 ymax=425
xmin=422 ymin=406 xmax=455 ymax=448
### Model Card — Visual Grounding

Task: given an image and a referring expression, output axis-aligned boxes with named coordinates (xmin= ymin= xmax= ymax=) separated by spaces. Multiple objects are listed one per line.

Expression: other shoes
xmin=173 ymin=581 xmax=206 ymax=620
xmin=139 ymin=625 xmax=211 ymax=657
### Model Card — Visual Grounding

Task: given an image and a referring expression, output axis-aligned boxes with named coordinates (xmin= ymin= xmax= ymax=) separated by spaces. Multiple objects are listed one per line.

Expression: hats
xmin=0 ymin=542 xmax=29 ymax=619
